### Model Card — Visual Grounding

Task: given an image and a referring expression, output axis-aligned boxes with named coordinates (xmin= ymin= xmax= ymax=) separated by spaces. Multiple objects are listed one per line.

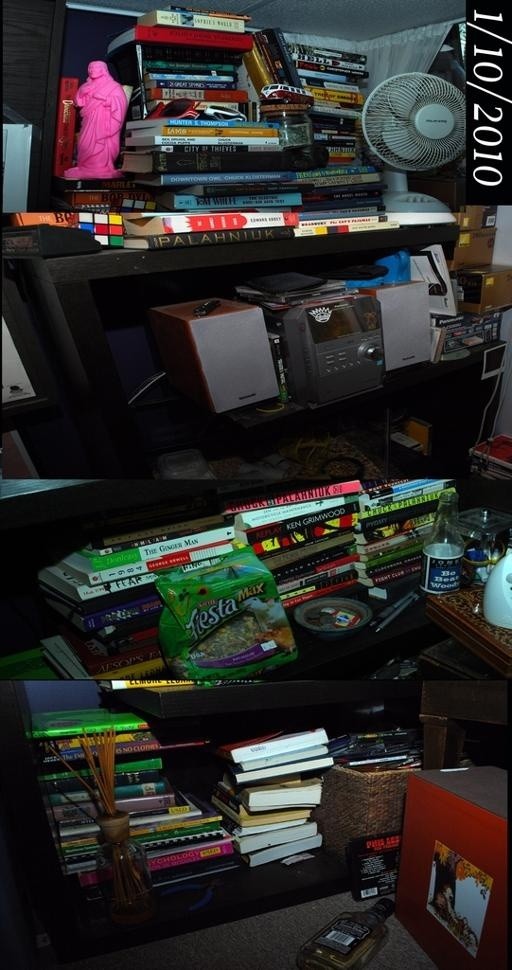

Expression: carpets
xmin=75 ymin=894 xmax=435 ymax=969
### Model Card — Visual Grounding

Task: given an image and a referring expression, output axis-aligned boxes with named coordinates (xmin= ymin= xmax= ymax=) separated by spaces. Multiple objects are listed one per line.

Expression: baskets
xmin=308 ymin=765 xmax=422 ymax=864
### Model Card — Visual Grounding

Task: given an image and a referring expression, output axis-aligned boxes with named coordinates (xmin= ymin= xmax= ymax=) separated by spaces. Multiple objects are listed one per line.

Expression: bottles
xmin=418 ymin=489 xmax=464 ymax=595
xmin=95 ymin=814 xmax=152 ymax=933
xmin=294 ymin=895 xmax=398 ymax=967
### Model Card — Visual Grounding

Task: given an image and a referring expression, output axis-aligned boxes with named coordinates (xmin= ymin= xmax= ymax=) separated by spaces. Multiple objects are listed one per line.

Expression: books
xmin=53 ymin=6 xmax=458 ymax=322
xmin=4 ymin=415 xmax=509 ymax=904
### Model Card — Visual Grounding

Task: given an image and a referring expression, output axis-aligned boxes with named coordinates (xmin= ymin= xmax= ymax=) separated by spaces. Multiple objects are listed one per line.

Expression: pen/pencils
xmin=439 ymin=767 xmax=470 ymax=772
xmin=369 ymin=591 xmax=422 ymax=632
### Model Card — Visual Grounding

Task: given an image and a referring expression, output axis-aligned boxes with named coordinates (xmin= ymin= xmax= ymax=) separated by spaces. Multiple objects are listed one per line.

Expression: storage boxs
xmin=448 ymin=204 xmax=510 ymax=320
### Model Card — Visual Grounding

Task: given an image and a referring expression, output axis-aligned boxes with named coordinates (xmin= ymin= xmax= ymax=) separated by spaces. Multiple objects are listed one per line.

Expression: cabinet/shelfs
xmin=8 ymin=224 xmax=462 ymax=480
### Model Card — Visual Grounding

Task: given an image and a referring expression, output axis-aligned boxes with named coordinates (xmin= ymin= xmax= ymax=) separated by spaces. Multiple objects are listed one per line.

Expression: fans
xmin=357 ymin=70 xmax=467 ymax=213
